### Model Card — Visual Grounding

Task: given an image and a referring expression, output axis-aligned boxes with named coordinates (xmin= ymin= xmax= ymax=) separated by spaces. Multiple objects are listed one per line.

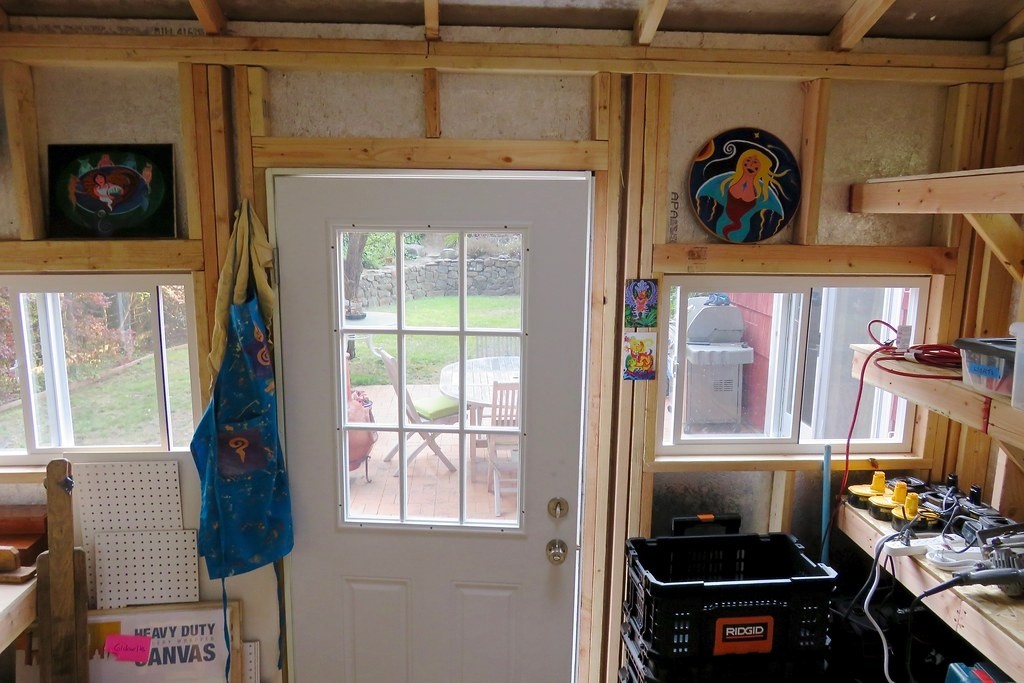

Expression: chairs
xmin=477 ymin=335 xmax=521 ymax=438
xmin=487 ymin=381 xmax=521 ymax=517
xmin=377 ymin=347 xmax=470 ymax=477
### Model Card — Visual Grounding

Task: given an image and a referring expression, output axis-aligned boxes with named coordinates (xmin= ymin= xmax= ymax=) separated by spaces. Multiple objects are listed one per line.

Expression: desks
xmin=439 ymin=357 xmax=519 ymax=483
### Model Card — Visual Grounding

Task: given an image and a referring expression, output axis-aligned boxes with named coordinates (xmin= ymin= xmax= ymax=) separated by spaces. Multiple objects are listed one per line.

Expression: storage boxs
xmin=953 ymin=319 xmax=1024 ymax=412
xmin=618 ymin=513 xmax=837 ymax=683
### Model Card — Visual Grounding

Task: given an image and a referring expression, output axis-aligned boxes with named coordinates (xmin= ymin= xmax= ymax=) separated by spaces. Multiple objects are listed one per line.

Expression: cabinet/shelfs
xmin=835 ymin=165 xmax=1024 ymax=682
